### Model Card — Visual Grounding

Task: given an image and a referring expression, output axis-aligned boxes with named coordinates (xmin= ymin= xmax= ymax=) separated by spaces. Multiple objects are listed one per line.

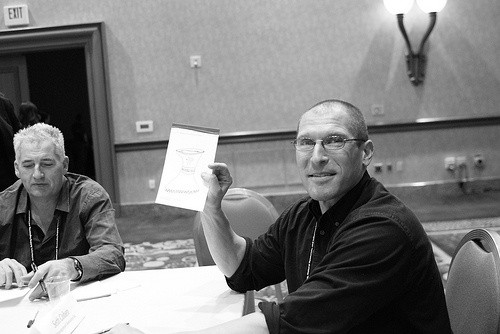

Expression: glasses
xmin=290 ymin=135 xmax=367 ymax=152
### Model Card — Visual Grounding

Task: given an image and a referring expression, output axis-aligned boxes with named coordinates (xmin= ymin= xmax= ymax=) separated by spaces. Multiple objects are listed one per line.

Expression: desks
xmin=0 ymin=263 xmax=264 ymax=334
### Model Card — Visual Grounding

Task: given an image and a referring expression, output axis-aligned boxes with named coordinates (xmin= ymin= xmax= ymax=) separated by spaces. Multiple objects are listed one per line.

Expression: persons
xmin=111 ymin=100 xmax=453 ymax=334
xmin=0 ymin=91 xmax=126 ymax=302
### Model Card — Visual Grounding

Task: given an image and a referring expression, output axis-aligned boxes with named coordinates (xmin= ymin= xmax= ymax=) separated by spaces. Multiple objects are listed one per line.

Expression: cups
xmin=44 ymin=276 xmax=71 ymax=303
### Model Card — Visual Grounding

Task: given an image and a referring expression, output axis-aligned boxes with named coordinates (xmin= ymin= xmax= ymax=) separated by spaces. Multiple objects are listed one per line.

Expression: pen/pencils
xmin=31 ymin=262 xmax=46 ymax=293
xmin=77 ymin=294 xmax=111 ymax=301
xmin=27 ymin=309 xmax=38 ymax=327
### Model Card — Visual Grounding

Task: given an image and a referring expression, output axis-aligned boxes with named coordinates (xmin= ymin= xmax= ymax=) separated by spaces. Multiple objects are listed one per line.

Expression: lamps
xmin=382 ymin=0 xmax=447 ymax=87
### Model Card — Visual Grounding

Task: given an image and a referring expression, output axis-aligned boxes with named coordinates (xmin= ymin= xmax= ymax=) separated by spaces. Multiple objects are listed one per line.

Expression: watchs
xmin=71 ymin=257 xmax=83 ymax=281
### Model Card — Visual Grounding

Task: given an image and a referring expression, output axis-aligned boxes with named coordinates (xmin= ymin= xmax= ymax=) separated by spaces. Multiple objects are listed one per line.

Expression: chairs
xmin=193 ymin=186 xmax=287 ymax=308
xmin=444 ymin=226 xmax=500 ymax=334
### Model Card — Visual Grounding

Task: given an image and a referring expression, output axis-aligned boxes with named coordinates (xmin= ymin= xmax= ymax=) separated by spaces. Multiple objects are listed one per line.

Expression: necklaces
xmin=28 ymin=209 xmax=60 ymax=263
xmin=306 ymin=222 xmax=318 ymax=280
